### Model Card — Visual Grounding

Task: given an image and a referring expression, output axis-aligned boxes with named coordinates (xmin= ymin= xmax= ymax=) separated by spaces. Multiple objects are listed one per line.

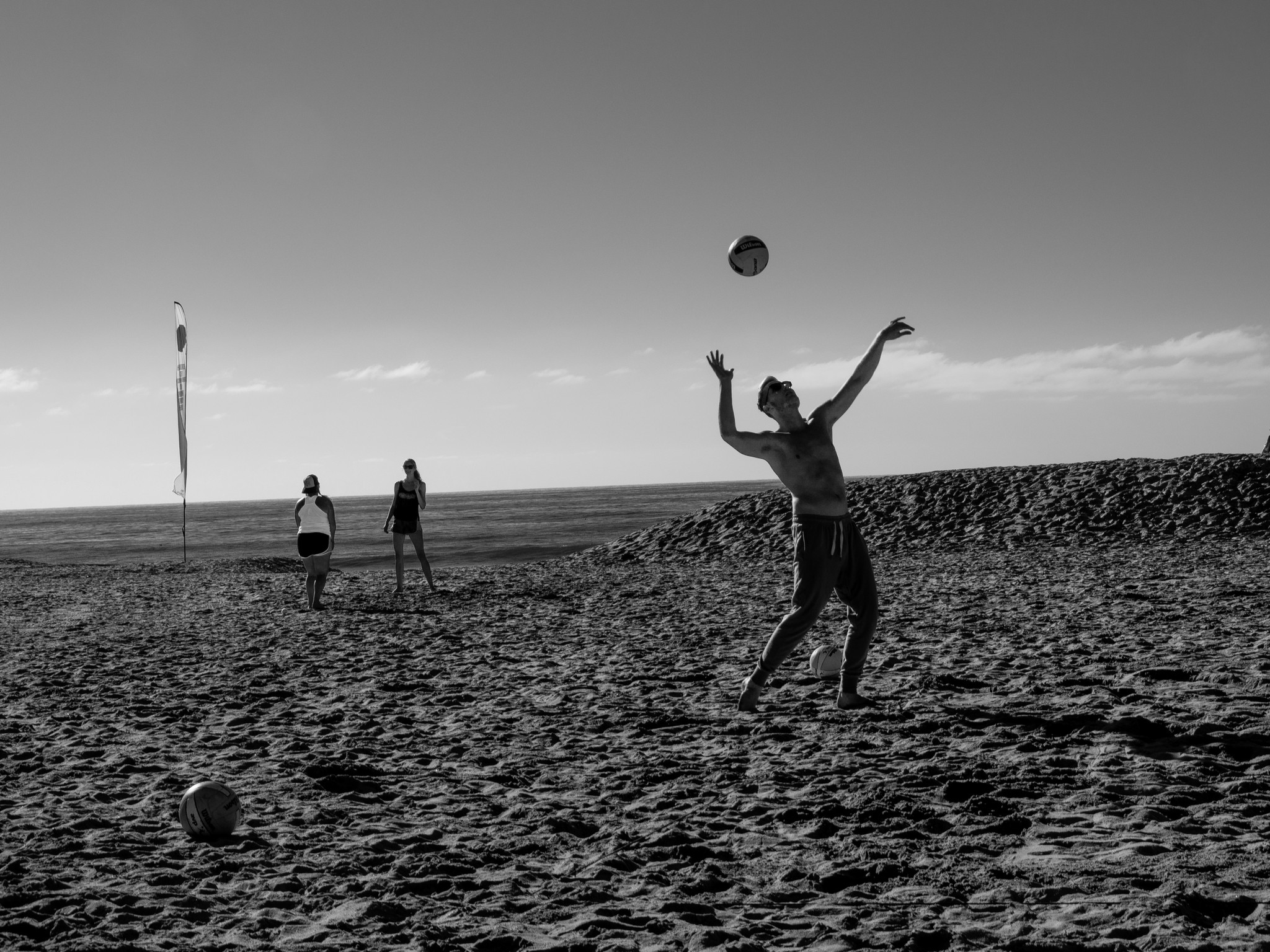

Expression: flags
xmin=172 ymin=300 xmax=188 ymax=502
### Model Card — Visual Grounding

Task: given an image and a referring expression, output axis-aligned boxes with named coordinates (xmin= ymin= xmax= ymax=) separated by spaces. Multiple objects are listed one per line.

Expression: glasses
xmin=403 ymin=465 xmax=415 ymax=469
xmin=764 ymin=380 xmax=793 ymax=408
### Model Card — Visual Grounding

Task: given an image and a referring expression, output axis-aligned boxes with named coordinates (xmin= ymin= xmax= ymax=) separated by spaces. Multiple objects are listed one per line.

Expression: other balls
xmin=281 ymin=608 xmax=288 ymax=614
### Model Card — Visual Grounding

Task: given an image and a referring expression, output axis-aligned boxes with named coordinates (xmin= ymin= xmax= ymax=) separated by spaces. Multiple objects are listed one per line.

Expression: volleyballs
xmin=727 ymin=234 xmax=770 ymax=277
xmin=178 ymin=780 xmax=242 ymax=841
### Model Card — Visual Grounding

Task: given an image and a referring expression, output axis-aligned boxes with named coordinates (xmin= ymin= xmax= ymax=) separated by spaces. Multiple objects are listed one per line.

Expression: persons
xmin=382 ymin=458 xmax=443 ymax=593
xmin=706 ymin=316 xmax=915 ymax=709
xmin=294 ymin=475 xmax=336 ymax=609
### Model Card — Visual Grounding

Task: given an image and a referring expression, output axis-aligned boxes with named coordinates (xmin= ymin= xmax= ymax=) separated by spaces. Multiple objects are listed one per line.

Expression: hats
xmin=302 ymin=474 xmax=319 ymax=493
